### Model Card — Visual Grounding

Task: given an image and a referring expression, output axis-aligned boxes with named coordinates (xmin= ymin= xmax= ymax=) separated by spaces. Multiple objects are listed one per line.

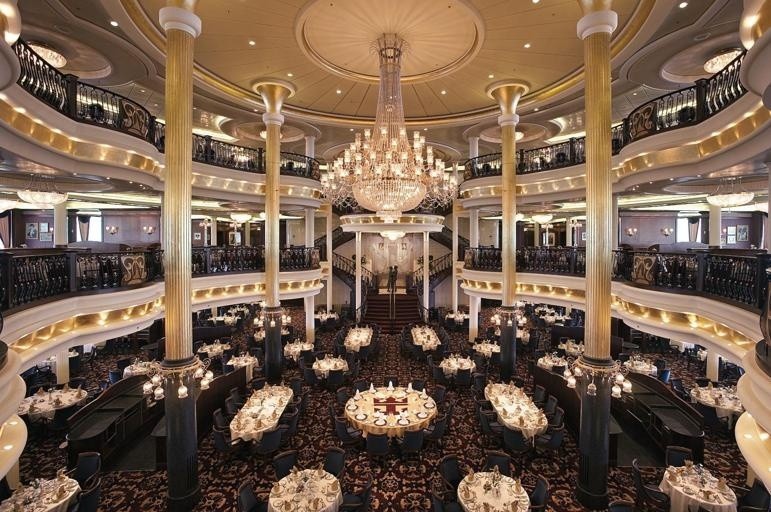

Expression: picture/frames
xmin=726 ymin=223 xmax=749 ymax=244
xmin=25 ymin=221 xmax=53 ymax=242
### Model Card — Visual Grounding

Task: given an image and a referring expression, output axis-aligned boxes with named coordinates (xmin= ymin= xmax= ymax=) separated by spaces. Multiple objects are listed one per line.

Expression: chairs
xmin=439 ymin=305 xmax=770 ymax=512
xmin=0 ymin=306 xmax=442 ymax=512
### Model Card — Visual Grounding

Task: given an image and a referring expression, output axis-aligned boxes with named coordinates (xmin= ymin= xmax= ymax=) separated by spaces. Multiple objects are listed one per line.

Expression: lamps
xmin=15 ymin=174 xmax=68 ymax=210
xmin=706 ymin=179 xmax=755 ymax=209
xmin=318 ymin=29 xmax=458 ymax=221
